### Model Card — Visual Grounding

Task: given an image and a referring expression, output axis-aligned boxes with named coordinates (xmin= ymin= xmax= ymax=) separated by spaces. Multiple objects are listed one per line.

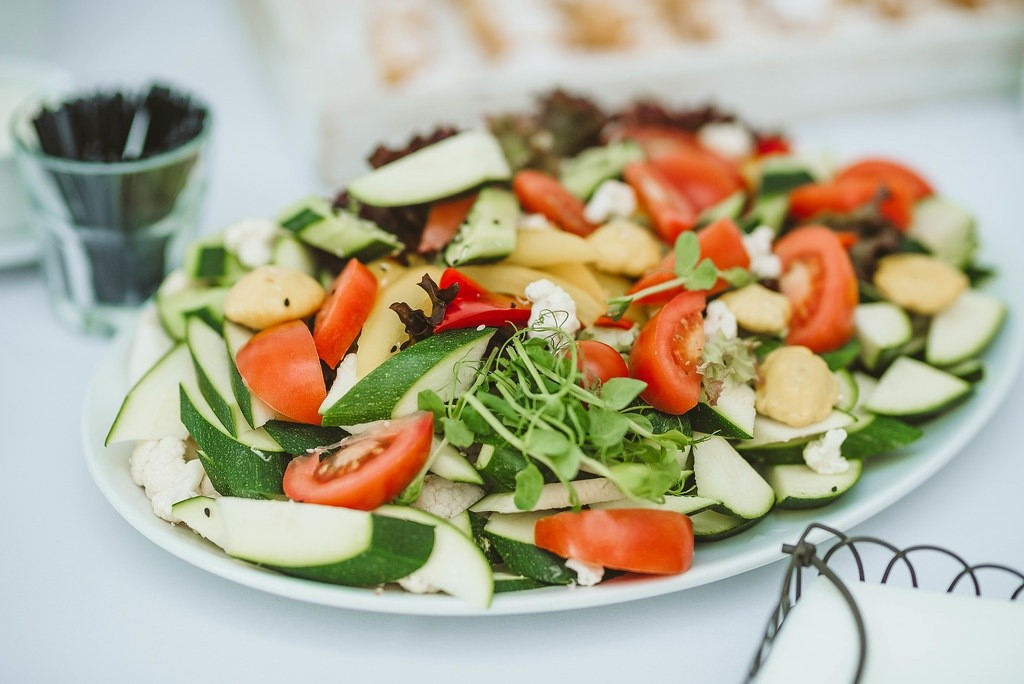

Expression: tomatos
xmin=234 ymin=124 xmax=935 ymax=575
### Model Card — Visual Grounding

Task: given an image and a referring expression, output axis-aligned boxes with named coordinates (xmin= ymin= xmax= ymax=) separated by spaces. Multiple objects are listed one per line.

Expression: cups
xmin=7 ymin=85 xmax=212 ymax=330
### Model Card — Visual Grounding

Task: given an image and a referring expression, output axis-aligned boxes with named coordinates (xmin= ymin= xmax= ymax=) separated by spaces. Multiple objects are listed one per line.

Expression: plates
xmin=84 ymin=107 xmax=1024 ymax=613
